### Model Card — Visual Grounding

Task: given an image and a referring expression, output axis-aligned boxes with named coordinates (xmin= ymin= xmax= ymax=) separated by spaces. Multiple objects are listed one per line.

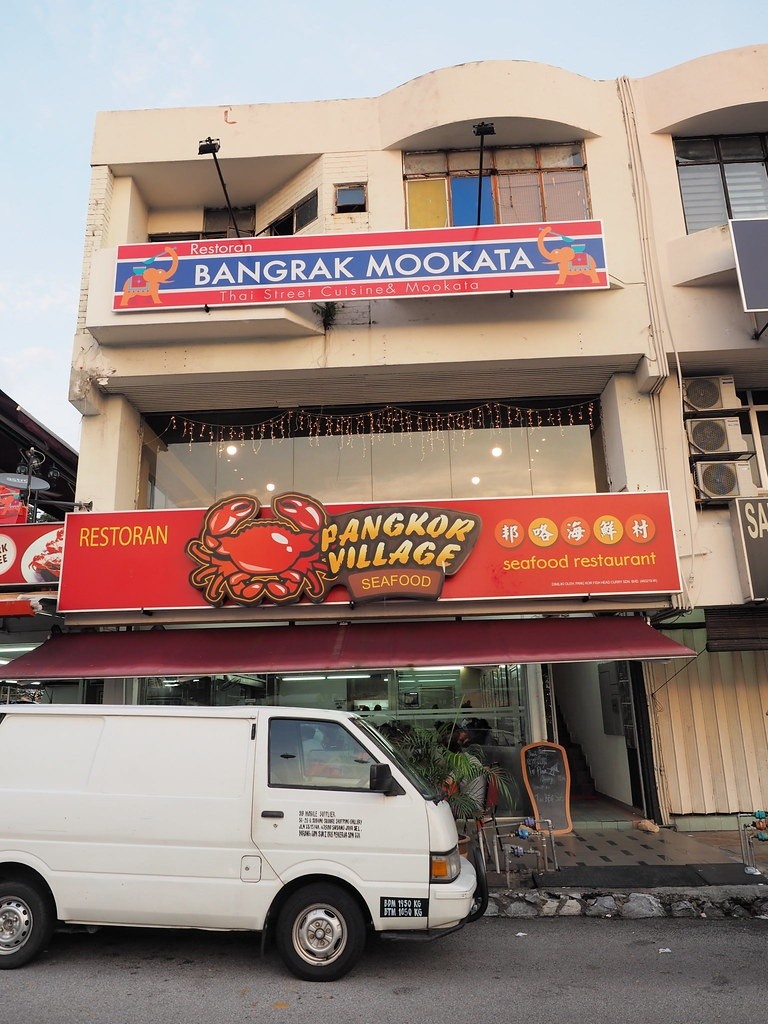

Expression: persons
xmin=360 ymin=699 xmax=492 ymax=820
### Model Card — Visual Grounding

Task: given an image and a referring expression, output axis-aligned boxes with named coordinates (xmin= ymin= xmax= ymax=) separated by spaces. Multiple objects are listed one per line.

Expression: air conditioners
xmin=682 ymin=375 xmax=758 ymax=505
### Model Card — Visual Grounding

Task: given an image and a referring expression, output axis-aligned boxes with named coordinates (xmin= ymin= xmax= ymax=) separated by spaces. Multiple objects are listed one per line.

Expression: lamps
xmin=198 ymin=137 xmax=220 ymax=155
xmin=472 ymin=122 xmax=496 ymax=135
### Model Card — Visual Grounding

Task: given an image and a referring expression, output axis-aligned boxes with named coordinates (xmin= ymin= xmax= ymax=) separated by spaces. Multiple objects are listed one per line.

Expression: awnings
xmin=0 ymin=616 xmax=700 ymax=683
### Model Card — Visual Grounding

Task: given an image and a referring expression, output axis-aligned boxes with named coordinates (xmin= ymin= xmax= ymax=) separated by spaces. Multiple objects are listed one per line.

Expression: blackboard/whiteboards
xmin=520 ymin=740 xmax=574 ymax=836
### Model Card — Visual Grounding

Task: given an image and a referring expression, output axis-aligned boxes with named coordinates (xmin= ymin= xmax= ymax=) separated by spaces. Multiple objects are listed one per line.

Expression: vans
xmin=0 ymin=700 xmax=490 ymax=983
